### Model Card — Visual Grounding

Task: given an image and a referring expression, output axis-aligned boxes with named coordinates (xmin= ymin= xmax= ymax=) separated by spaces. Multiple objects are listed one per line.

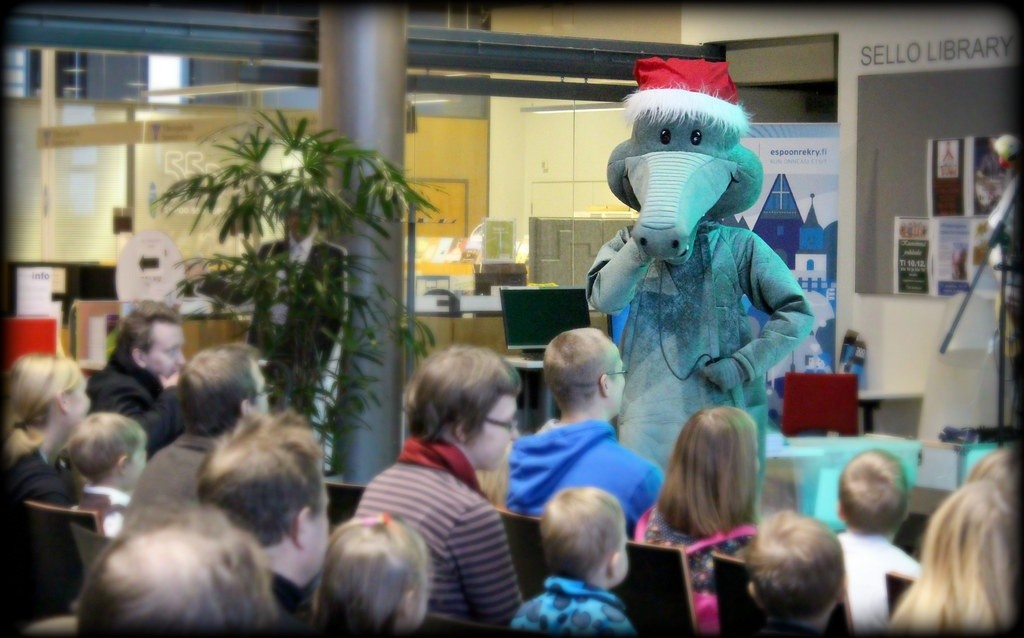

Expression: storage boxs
xmin=893 ymin=215 xmax=1022 ymax=298
xmin=927 ymin=136 xmax=1008 ymax=219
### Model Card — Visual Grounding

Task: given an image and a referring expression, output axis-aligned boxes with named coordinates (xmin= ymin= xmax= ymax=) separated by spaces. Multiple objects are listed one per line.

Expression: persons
xmin=1 ymin=302 xmax=1018 ymax=635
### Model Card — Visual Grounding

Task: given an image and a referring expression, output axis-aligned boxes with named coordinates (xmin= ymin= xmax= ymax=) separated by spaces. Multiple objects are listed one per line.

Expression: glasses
xmin=606 ymin=367 xmax=628 ymax=376
xmin=485 ymin=418 xmax=518 ymax=434
xmin=255 ymin=383 xmax=275 ymax=395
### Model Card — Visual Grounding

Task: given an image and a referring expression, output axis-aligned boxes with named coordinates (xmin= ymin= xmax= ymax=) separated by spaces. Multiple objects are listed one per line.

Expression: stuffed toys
xmin=584 ymin=51 xmax=819 ymax=522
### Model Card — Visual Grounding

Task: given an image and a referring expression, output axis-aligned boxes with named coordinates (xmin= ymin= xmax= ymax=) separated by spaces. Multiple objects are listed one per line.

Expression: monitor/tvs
xmin=499 ymin=285 xmax=591 ymax=349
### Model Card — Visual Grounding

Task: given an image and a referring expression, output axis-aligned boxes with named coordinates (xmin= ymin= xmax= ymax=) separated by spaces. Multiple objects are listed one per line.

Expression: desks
xmin=504 ymin=357 xmax=958 ymax=516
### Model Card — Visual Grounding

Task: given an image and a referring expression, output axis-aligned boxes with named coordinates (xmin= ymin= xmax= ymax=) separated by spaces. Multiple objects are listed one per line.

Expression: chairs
xmin=20 ymin=481 xmax=915 ymax=638
xmin=781 ymin=371 xmax=858 ymax=438
